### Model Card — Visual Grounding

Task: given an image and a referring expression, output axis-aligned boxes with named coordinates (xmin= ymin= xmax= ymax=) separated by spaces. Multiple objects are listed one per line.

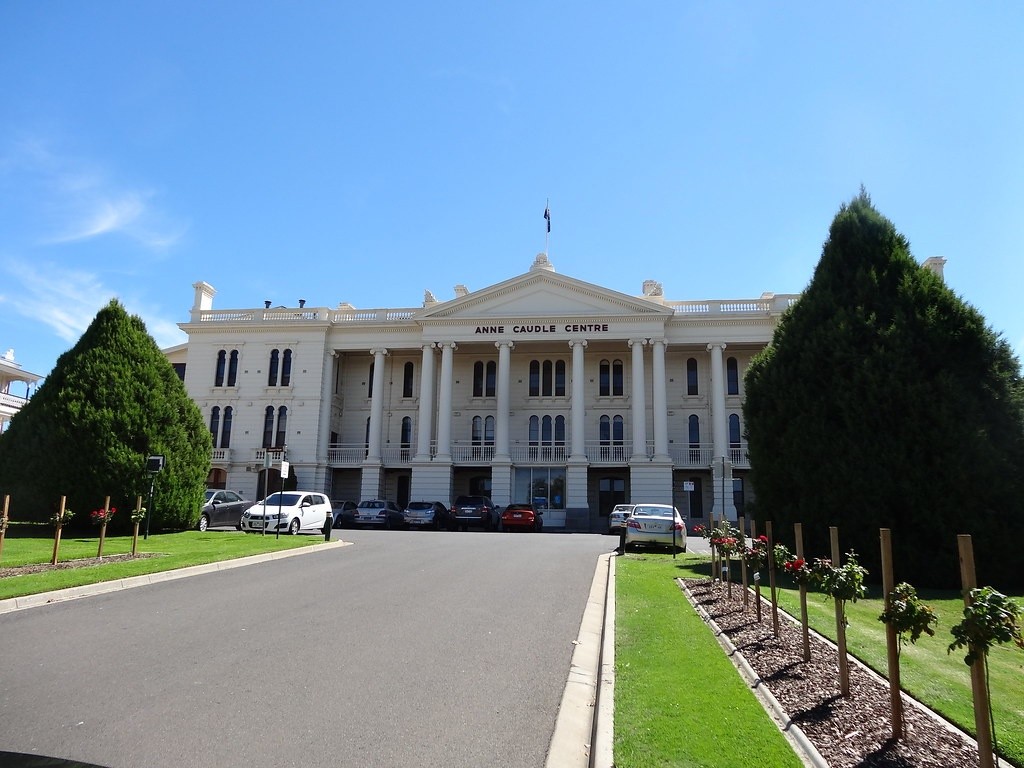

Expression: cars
xmin=240 ymin=491 xmax=334 ymax=534
xmin=400 ymin=500 xmax=452 ymax=531
xmin=623 ymin=504 xmax=687 ymax=553
xmin=608 ymin=504 xmax=643 ymax=533
xmin=354 ymin=500 xmax=404 ymax=531
xmin=500 ymin=502 xmax=544 ymax=533
xmin=194 ymin=489 xmax=255 ymax=532
xmin=329 ymin=500 xmax=358 ymax=528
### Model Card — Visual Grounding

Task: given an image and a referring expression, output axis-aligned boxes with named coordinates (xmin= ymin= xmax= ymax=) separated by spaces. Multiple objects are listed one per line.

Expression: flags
xmin=544 ymin=208 xmax=550 ymax=232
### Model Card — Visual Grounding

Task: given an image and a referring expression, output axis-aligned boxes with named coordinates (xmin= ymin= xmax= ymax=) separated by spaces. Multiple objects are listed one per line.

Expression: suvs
xmin=450 ymin=495 xmax=501 ymax=532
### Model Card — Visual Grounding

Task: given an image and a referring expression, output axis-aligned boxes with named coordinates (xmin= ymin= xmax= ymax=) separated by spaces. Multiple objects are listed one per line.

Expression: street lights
xmin=277 ymin=444 xmax=288 ymax=538
xmin=144 ymin=455 xmax=166 ymax=540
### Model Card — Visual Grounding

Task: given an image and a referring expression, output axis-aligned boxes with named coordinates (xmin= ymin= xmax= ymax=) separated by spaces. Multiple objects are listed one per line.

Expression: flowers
xmin=88 ymin=507 xmax=117 ymax=527
xmin=131 ymin=507 xmax=147 ymax=524
xmin=743 ymin=535 xmax=769 ymax=569
xmin=707 ymin=528 xmax=747 ymax=558
xmin=773 ymin=544 xmax=811 ymax=584
xmin=693 ymin=524 xmax=709 ymax=539
